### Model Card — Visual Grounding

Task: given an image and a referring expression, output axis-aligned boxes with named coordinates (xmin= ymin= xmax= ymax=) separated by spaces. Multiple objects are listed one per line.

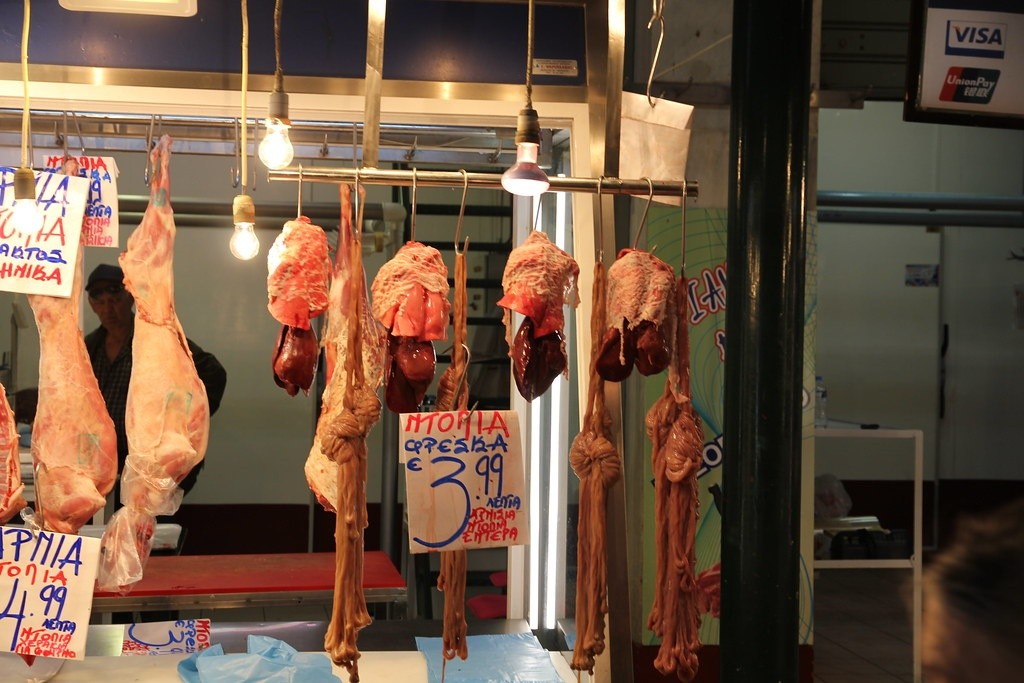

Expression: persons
xmin=915 ymin=506 xmax=1024 ymax=683
xmin=83 ymin=262 xmax=227 ymax=556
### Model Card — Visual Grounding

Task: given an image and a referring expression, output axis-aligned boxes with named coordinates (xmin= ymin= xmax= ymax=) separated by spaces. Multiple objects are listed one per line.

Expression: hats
xmin=85 ymin=261 xmax=126 ymax=294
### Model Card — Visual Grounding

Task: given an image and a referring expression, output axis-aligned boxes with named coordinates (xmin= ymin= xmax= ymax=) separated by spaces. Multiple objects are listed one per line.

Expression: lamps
xmin=12 ymin=0 xmax=46 ymax=235
xmin=499 ymin=0 xmax=552 ymax=196
xmin=544 ymin=172 xmax=567 ymax=630
xmin=528 ymin=196 xmax=542 ymax=633
xmin=229 ymin=0 xmax=260 ymax=261
xmin=258 ymin=0 xmax=295 ymax=170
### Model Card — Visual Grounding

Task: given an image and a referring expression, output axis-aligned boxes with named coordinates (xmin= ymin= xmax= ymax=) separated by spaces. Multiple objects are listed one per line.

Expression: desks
xmin=89 ymin=547 xmax=411 ymax=628
xmin=0 ymin=617 xmax=556 ymax=682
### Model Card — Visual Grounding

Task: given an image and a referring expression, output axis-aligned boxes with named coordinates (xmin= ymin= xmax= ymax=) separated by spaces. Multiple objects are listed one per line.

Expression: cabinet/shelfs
xmin=814 ymin=419 xmax=923 ymax=683
xmin=812 ymin=99 xmax=1023 ymax=548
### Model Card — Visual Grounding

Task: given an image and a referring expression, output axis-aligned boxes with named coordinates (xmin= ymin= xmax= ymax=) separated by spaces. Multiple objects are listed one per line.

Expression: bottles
xmin=815 ymin=376 xmax=828 ymax=428
